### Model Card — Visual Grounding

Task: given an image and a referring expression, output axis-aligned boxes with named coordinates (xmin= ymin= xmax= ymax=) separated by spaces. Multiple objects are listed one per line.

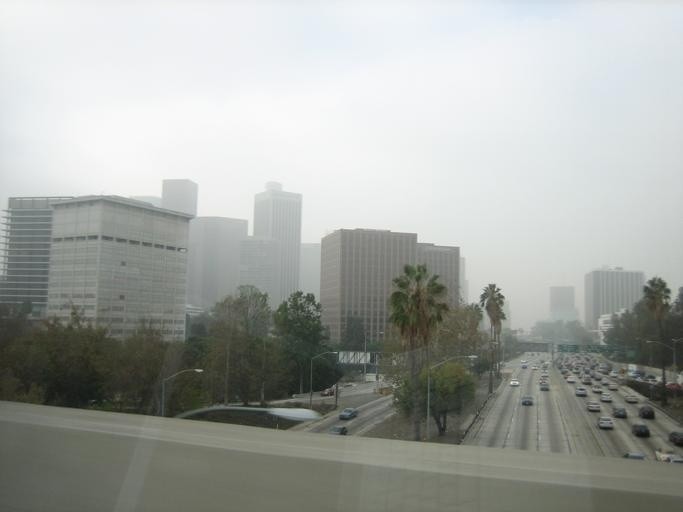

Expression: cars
xmin=339 ymin=407 xmax=358 ymax=420
xmin=320 ymin=388 xmax=334 ymax=397
xmin=510 ymin=359 xmax=550 ymax=405
xmin=556 ymin=348 xmax=682 ymax=464
xmin=328 ymin=424 xmax=347 ymax=435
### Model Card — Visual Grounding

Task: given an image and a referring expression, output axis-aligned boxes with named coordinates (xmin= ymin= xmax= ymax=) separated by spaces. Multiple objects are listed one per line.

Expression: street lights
xmin=161 ymin=368 xmax=204 ymax=416
xmin=645 ymin=339 xmax=680 ymax=407
xmin=363 ymin=330 xmax=384 ymax=385
xmin=426 ymin=354 xmax=479 ymax=441
xmin=309 ymin=350 xmax=339 ymax=410
